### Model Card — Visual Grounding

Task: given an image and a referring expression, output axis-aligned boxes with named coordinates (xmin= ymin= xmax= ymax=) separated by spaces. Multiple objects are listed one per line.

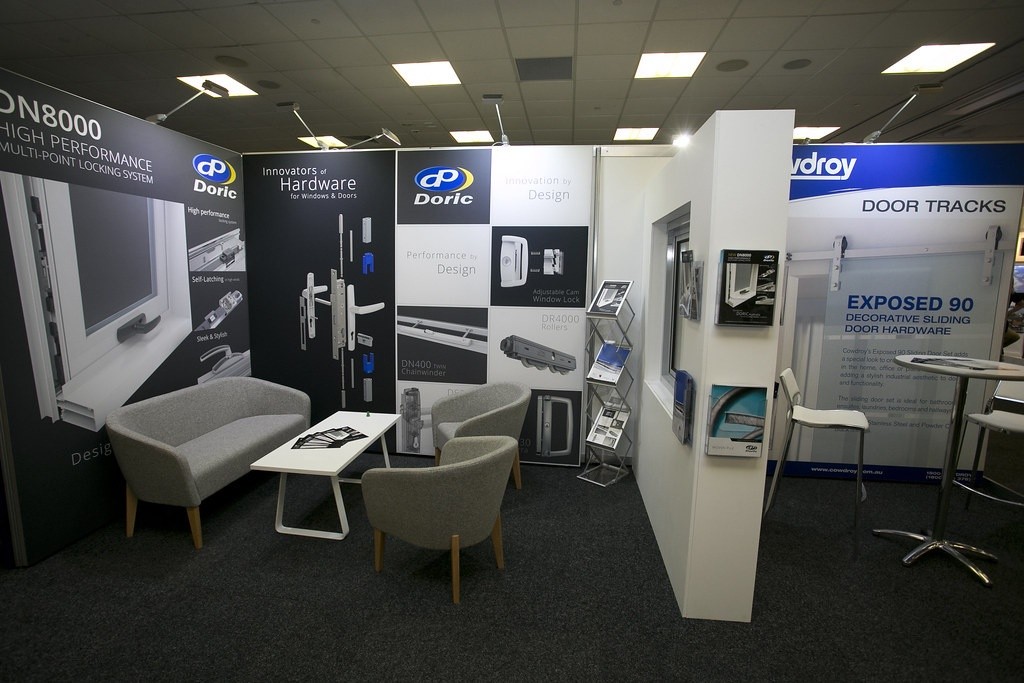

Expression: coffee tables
xmin=250 ymin=410 xmax=403 ymax=541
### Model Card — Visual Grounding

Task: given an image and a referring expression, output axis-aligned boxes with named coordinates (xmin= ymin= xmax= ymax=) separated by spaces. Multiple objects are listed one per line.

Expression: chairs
xmin=361 ymin=435 xmax=519 ymax=603
xmin=431 ymin=382 xmax=533 ymax=490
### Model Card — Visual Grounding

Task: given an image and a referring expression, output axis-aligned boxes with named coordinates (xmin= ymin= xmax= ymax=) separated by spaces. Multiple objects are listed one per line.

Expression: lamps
xmin=277 ymin=101 xmax=329 ymax=151
xmin=340 ymin=127 xmax=402 ymax=149
xmin=481 ymin=94 xmax=512 ymax=146
xmin=145 ymin=80 xmax=230 ymax=125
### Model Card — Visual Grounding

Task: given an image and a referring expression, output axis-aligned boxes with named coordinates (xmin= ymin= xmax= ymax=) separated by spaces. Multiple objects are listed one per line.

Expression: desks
xmin=873 ymin=354 xmax=1024 ymax=587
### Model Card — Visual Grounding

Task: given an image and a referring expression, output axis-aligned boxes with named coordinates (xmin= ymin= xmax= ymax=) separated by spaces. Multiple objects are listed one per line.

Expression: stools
xmin=952 ymin=380 xmax=1024 ymax=512
xmin=764 ymin=367 xmax=870 ymax=550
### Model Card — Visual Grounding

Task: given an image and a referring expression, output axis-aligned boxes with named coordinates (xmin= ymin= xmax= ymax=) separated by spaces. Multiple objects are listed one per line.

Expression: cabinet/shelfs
xmin=575 ymin=278 xmax=637 ymax=488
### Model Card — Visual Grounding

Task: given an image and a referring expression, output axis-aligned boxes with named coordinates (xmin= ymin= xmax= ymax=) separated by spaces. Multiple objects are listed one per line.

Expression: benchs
xmin=104 ymin=376 xmax=311 ymax=548
